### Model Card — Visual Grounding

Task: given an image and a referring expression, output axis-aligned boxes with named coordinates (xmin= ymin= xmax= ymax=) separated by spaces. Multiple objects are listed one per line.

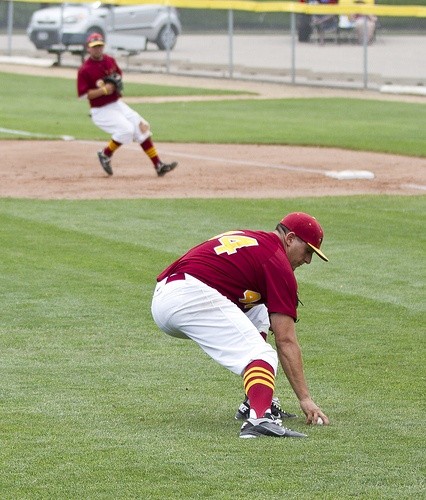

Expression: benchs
xmin=49 ymin=32 xmax=148 ymax=72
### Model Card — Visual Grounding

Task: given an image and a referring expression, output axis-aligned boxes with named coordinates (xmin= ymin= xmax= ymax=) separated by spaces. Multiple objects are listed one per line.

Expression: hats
xmin=280 ymin=212 xmax=329 ymax=262
xmin=87 ymin=33 xmax=104 ymax=48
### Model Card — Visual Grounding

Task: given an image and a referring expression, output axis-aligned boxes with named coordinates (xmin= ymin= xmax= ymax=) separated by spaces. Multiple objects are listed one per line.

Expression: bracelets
xmin=102 ymin=87 xmax=108 ymax=95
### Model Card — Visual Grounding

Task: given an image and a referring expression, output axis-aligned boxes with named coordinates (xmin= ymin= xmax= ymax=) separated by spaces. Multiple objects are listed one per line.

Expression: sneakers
xmin=155 ymin=161 xmax=177 ymax=176
xmin=97 ymin=151 xmax=114 ymax=176
xmin=235 ymin=394 xmax=299 ymax=420
xmin=239 ymin=411 xmax=308 ymax=440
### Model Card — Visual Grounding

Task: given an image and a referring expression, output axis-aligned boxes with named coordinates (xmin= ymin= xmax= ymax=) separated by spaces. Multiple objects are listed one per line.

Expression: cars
xmin=27 ymin=1 xmax=183 ymax=54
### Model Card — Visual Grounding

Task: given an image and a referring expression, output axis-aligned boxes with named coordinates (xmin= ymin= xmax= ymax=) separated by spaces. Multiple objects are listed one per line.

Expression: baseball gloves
xmin=103 ymin=72 xmax=124 ymax=93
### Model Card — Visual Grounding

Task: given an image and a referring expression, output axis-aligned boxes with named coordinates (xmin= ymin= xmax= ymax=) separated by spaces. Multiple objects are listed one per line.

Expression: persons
xmin=77 ymin=33 xmax=177 ymax=177
xmin=297 ymin=0 xmax=338 ymax=42
xmin=151 ymin=212 xmax=329 ymax=438
xmin=337 ymin=0 xmax=377 ymax=45
